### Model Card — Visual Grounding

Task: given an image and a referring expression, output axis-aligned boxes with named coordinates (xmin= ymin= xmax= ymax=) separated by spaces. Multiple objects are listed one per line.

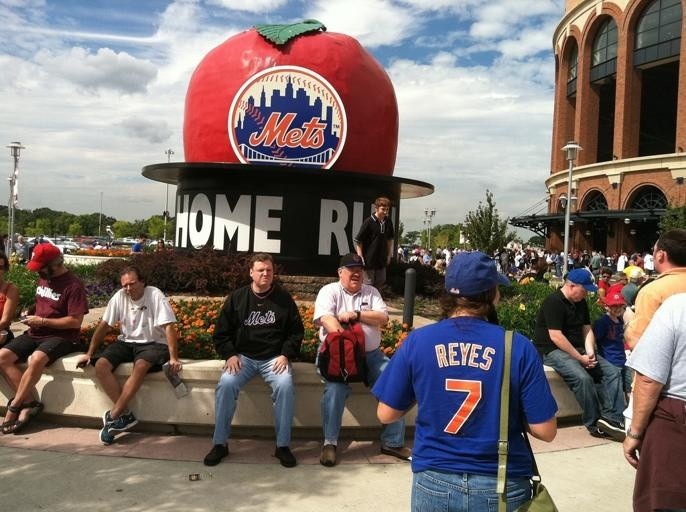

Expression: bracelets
xmin=627 ymin=427 xmax=643 ymax=439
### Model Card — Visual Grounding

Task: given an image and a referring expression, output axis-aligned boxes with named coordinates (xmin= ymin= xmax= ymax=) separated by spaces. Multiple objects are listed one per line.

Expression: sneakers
xmin=591 ymin=427 xmax=615 ymax=440
xmin=274 ymin=444 xmax=296 ymax=467
xmin=597 ymin=416 xmax=627 ymax=442
xmin=99 ymin=409 xmax=115 ymax=445
xmin=204 ymin=443 xmax=229 ymax=465
xmin=107 ymin=409 xmax=138 ymax=436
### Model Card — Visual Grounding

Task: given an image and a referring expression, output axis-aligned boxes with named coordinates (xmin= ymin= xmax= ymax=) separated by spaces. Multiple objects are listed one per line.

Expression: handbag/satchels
xmin=497 ymin=331 xmax=557 ymax=511
xmin=544 ymin=266 xmax=552 ymax=280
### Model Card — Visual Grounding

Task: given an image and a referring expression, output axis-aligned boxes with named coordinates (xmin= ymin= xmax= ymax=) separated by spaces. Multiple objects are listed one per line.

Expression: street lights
xmin=162 ymin=147 xmax=174 ymax=240
xmin=5 ymin=140 xmax=26 ymax=261
xmin=97 ymin=190 xmax=103 ymax=235
xmin=422 ymin=207 xmax=436 ymax=253
xmin=560 ymin=140 xmax=584 ymax=282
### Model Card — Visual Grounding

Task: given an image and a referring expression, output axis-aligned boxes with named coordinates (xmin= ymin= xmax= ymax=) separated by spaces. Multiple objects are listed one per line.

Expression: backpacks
xmin=318 ymin=317 xmax=372 ymax=387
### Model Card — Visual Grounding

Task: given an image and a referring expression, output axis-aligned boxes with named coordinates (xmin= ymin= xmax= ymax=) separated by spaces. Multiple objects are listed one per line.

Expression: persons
xmin=592 ymin=292 xmax=632 ymax=401
xmin=534 ymin=269 xmax=627 ymax=440
xmin=76 ymin=266 xmax=182 ymax=446
xmin=398 ymin=240 xmax=655 ymax=305
xmin=371 ymin=251 xmax=559 ymax=512
xmin=628 ymin=229 xmax=686 ymax=346
xmin=312 ymin=253 xmax=411 ymax=467
xmin=623 ymin=293 xmax=686 ymax=512
xmin=0 ymin=233 xmax=89 ymax=433
xmin=204 ymin=254 xmax=304 ymax=467
xmin=353 ymin=197 xmax=394 ymax=284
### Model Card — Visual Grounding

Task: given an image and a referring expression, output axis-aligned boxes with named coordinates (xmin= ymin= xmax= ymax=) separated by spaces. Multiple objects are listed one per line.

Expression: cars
xmin=19 ymin=234 xmax=173 ymax=254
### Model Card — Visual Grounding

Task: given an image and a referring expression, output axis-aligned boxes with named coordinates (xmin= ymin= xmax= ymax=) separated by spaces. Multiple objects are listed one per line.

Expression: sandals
xmin=1 ymin=398 xmax=22 ymax=434
xmin=11 ymin=401 xmax=44 ymax=433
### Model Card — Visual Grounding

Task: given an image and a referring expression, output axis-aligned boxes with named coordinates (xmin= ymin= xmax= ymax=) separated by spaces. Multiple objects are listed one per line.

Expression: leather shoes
xmin=320 ymin=442 xmax=337 ymax=467
xmin=381 ymin=444 xmax=413 ymax=463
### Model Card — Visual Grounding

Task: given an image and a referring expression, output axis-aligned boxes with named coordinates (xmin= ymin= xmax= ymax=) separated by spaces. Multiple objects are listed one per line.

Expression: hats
xmin=340 ymin=253 xmax=366 ymax=270
xmin=629 ymin=269 xmax=646 ymax=279
xmin=567 ymin=268 xmax=598 ymax=293
xmin=25 ymin=243 xmax=60 ymax=271
xmin=604 ymin=293 xmax=626 ymax=306
xmin=445 ymin=251 xmax=511 ymax=296
